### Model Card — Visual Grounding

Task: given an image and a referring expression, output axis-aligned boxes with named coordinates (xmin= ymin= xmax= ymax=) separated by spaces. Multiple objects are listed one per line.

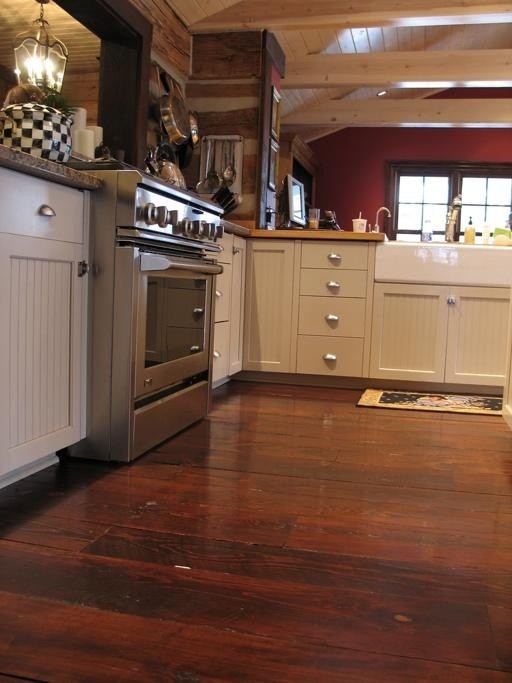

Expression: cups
xmin=308 ymin=208 xmax=320 ymax=230
xmin=352 ymin=219 xmax=367 ymax=233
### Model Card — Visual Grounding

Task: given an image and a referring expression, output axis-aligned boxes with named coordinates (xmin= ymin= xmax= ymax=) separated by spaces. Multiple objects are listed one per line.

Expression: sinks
xmin=373 ymin=241 xmax=512 ymax=288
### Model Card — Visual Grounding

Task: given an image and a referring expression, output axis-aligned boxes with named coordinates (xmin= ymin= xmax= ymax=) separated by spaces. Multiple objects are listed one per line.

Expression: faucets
xmin=445 ymin=197 xmax=461 ymax=242
xmin=376 ymin=207 xmax=391 ymax=225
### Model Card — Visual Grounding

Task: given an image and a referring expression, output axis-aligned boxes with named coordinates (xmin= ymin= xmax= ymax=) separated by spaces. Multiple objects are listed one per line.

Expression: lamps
xmin=12 ymin=0 xmax=69 ymax=94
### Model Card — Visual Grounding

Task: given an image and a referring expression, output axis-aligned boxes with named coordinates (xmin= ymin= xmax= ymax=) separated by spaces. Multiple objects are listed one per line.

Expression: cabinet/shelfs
xmin=294 ymin=240 xmax=376 ymax=390
xmin=0 ymin=165 xmax=92 ymax=496
xmin=243 ymin=238 xmax=299 ymax=387
xmin=205 ymin=231 xmax=248 ymax=388
xmin=369 ymin=281 xmax=510 ymax=396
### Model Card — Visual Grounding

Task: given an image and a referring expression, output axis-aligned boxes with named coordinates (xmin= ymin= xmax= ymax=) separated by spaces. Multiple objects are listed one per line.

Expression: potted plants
xmin=1 ymin=90 xmax=81 ymax=165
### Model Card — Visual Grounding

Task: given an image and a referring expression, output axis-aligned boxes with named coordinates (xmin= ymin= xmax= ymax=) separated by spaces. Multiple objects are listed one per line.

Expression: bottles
xmin=421 ymin=220 xmax=433 ymax=243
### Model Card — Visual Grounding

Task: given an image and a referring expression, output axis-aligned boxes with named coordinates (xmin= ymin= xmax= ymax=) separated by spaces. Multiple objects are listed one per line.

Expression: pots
xmin=159 ymin=72 xmax=199 ymax=168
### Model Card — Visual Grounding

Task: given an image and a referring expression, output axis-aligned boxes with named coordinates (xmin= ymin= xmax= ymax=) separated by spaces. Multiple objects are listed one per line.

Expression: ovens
xmin=109 ymin=185 xmax=225 ymax=462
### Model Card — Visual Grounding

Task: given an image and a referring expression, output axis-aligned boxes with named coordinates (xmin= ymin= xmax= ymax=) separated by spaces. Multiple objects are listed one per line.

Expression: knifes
xmin=209 ymin=185 xmax=239 ymax=218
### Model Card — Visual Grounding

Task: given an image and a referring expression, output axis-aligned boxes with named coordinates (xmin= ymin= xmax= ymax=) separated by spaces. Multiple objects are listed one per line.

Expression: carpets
xmin=356 ymin=387 xmax=502 ymax=417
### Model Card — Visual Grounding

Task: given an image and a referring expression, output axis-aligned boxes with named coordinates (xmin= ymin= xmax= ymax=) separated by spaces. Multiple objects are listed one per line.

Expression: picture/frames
xmin=285 ymin=174 xmax=308 ymax=226
xmin=268 ymin=137 xmax=281 ymax=192
xmin=270 ymin=84 xmax=282 ymax=145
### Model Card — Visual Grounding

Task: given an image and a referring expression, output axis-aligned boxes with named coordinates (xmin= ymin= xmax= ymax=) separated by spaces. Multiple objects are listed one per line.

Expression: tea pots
xmin=144 ymin=142 xmax=187 ymax=191
